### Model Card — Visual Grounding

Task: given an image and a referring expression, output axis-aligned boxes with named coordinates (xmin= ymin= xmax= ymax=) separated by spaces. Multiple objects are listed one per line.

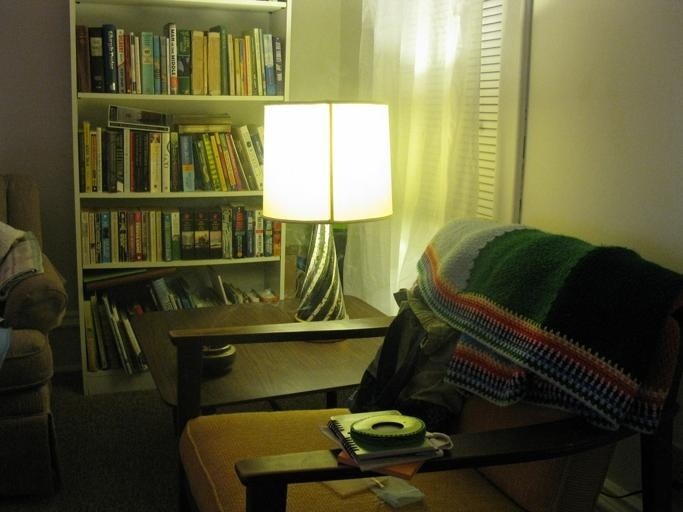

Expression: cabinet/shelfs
xmin=69 ymin=0 xmax=293 ymax=399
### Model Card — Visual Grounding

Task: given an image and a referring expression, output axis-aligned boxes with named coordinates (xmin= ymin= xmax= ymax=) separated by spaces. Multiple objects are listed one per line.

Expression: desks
xmin=128 ymin=291 xmax=396 ymax=457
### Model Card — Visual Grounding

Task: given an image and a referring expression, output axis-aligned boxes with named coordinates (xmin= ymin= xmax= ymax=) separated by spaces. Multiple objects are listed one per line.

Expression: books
xmin=75 ymin=22 xmax=283 ymax=96
xmin=81 ymin=201 xmax=281 ymax=264
xmin=83 ymin=267 xmax=280 ymax=376
xmin=351 ymin=447 xmax=444 ymax=472
xmin=320 ymin=472 xmax=392 ymax=498
xmin=328 ymin=409 xmax=437 ymax=461
xmin=79 ymin=105 xmax=264 ymax=194
xmin=337 ymin=450 xmax=425 ymax=479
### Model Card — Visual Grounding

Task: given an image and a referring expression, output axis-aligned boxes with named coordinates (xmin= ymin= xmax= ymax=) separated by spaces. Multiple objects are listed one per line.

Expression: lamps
xmin=259 ymin=98 xmax=396 ymax=344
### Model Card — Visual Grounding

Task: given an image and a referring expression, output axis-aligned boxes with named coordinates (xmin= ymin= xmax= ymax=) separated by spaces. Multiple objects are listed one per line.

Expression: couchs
xmin=170 ymin=212 xmax=683 ymax=510
xmin=0 ymin=169 xmax=70 ymax=501
